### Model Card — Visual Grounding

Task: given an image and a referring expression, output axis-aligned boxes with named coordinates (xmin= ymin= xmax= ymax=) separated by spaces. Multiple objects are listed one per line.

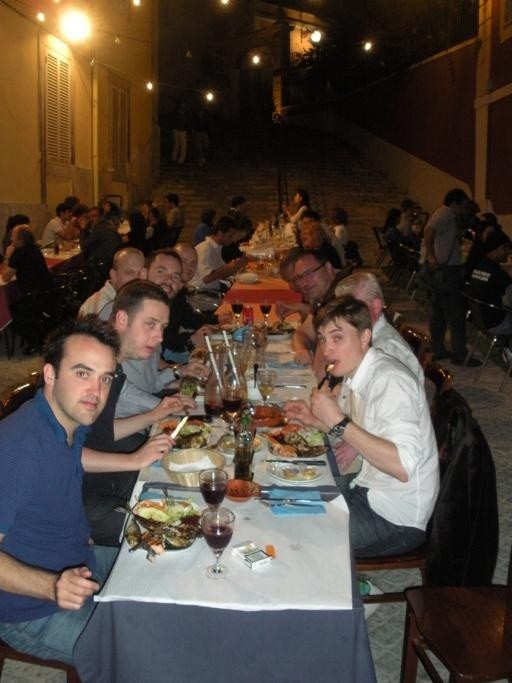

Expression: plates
xmin=125 ymin=499 xmax=199 ymax=551
xmin=160 ymin=407 xmax=303 ymax=457
xmin=236 ymin=274 xmax=259 ymax=283
xmin=227 ymin=478 xmax=259 ymax=501
xmin=268 ymin=428 xmax=325 ymax=483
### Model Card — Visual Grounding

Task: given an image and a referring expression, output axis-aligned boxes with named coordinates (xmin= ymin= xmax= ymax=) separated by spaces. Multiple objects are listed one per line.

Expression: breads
xmin=281 ymin=468 xmax=318 ymax=480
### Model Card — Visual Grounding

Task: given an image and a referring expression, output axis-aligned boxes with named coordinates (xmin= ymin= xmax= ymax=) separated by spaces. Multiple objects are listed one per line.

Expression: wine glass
xmin=182 ymin=299 xmax=295 ymax=422
xmin=198 ymin=469 xmax=236 ymax=577
xmin=258 ymin=255 xmax=280 ymax=279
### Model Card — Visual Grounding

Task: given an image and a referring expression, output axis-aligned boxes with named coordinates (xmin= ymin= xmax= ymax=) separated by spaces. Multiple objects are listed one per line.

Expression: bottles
xmin=233 ymin=408 xmax=254 ymax=482
xmin=53 ymin=235 xmax=60 ymax=255
xmin=267 ymin=212 xmax=279 ymax=239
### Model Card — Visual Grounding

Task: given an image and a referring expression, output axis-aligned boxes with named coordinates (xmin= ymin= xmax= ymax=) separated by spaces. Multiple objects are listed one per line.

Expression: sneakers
xmin=450 ymin=353 xmax=483 ymax=368
xmin=362 ymin=580 xmax=386 ymax=619
xmin=428 ymin=347 xmax=456 ymax=360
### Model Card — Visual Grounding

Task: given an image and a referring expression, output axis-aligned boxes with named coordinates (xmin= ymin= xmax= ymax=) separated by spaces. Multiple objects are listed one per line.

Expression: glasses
xmin=292 ymin=261 xmax=327 ymax=285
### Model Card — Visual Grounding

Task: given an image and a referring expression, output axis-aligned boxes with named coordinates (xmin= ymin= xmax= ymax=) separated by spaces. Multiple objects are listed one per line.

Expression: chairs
xmin=371 ymin=224 xmax=509 ymax=400
xmin=357 ymin=552 xmax=511 ymax=683
xmin=186 ymin=287 xmax=224 ymax=313
xmin=1 ymin=239 xmax=110 ymax=360
xmin=1 ymin=371 xmax=80 ymax=682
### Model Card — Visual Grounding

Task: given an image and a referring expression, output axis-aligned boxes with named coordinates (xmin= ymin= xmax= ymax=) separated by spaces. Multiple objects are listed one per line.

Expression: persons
xmin=171 ymin=100 xmax=193 ymax=167
xmin=191 ymin=105 xmax=209 ymax=168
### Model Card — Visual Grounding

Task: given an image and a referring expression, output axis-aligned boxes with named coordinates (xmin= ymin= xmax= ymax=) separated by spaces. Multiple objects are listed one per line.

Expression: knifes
xmin=254 ymin=498 xmax=328 ymax=505
xmin=262 ymin=459 xmax=325 ymax=465
xmin=130 ymin=515 xmax=177 ymax=553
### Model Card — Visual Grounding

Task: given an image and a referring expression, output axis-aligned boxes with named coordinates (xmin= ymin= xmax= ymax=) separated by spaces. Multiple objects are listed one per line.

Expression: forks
xmin=161 ymin=484 xmax=174 ymax=506
xmin=318 ymin=361 xmax=334 ymax=389
xmin=113 ymin=505 xmax=159 ymax=531
